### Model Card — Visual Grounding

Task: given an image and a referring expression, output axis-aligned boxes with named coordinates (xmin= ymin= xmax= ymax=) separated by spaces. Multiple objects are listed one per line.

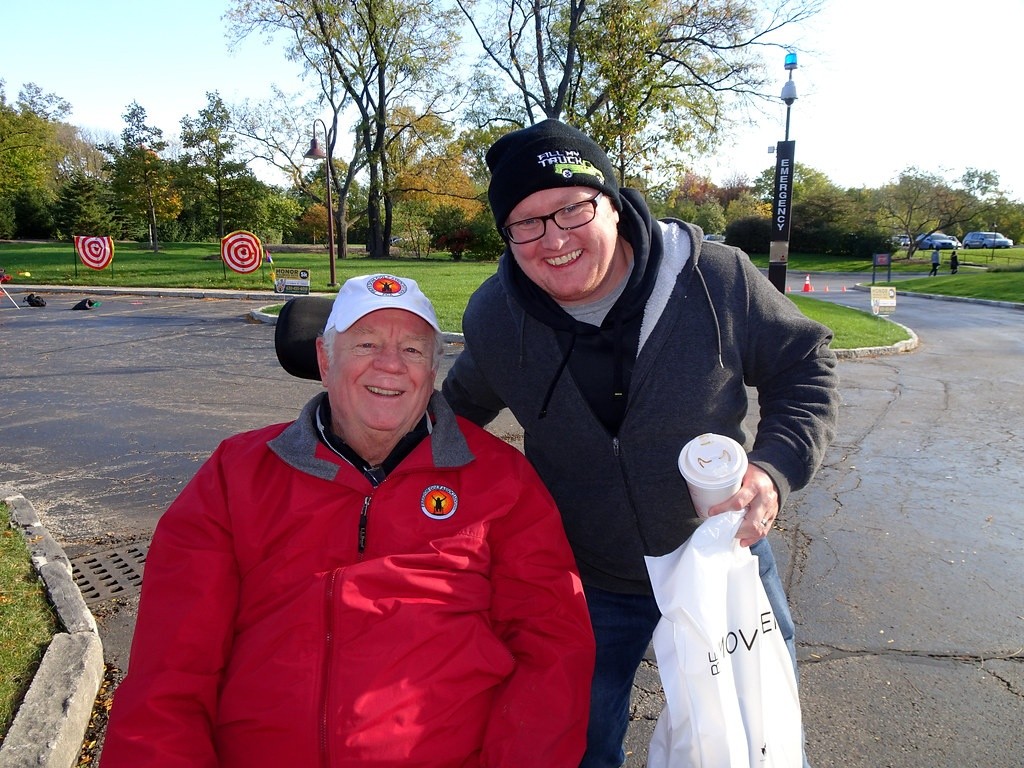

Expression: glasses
xmin=502 ymin=190 xmax=602 ymax=244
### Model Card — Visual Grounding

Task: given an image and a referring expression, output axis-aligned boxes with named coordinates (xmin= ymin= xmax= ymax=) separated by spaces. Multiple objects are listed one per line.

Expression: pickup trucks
xmin=962 ymin=231 xmax=1013 ymax=249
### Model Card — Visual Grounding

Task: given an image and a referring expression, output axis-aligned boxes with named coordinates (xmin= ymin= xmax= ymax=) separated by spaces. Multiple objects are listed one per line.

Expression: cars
xmin=918 ymin=232 xmax=953 ymax=250
xmin=948 ymin=235 xmax=962 ymax=249
xmin=897 ymin=234 xmax=911 ymax=246
xmin=705 ymin=234 xmax=726 ymax=243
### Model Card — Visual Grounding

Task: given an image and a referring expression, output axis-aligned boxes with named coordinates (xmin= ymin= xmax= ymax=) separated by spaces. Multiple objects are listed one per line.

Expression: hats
xmin=323 ymin=273 xmax=442 ymax=335
xmin=485 ymin=118 xmax=622 ymax=246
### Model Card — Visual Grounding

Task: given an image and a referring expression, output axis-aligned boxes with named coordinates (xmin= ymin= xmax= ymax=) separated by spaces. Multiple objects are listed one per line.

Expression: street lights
xmin=304 ymin=117 xmax=340 ymax=287
xmin=759 ymin=52 xmax=801 ymax=292
xmin=991 ymin=221 xmax=998 ymax=260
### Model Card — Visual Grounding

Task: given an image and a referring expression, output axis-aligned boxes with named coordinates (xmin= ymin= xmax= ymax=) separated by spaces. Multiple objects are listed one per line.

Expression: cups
xmin=678 ymin=432 xmax=748 ymax=519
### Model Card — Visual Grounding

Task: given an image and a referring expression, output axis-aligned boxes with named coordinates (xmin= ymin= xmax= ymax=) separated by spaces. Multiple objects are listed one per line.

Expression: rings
xmin=762 ymin=520 xmax=767 ymax=526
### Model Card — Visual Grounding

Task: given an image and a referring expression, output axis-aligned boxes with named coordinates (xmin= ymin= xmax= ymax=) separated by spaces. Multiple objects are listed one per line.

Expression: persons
xmin=441 ymin=120 xmax=837 ymax=768
xmin=951 ymin=251 xmax=959 ymax=274
xmin=929 ymin=246 xmax=940 ymax=276
xmin=98 ymin=274 xmax=596 ymax=768
